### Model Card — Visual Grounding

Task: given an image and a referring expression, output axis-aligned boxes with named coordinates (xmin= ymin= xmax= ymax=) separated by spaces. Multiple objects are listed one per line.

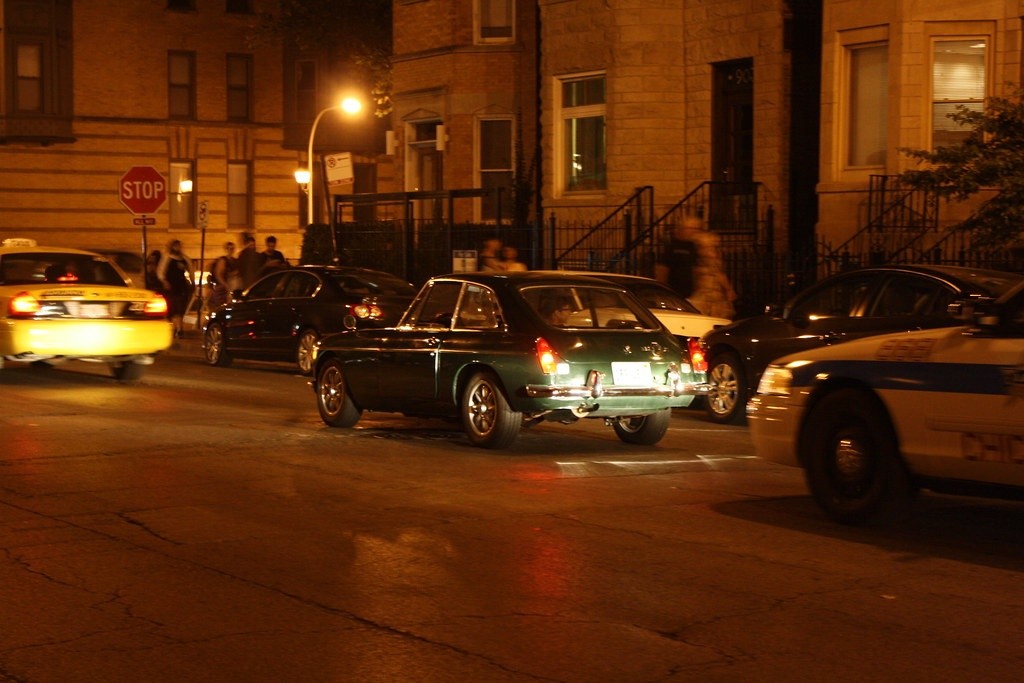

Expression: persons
xmin=543 ymin=283 xmax=572 ymax=326
xmin=656 ymin=217 xmax=701 ymax=297
xmin=45 ymin=266 xmax=61 ymax=282
xmin=480 ymin=236 xmax=506 ymax=276
xmin=238 ymin=237 xmax=260 ymax=289
xmin=216 ymin=241 xmax=240 ymax=295
xmin=502 ymin=247 xmax=528 ymax=272
xmin=258 ymin=236 xmax=289 ymax=276
xmin=687 ymin=231 xmax=736 ymax=318
xmin=156 ymin=240 xmax=196 ymax=339
xmin=146 ymin=250 xmax=164 ymax=293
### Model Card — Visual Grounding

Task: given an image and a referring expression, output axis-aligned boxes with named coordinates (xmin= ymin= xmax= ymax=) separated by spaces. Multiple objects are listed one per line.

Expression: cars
xmin=306 ymin=269 xmax=734 ymax=451
xmin=0 ymin=237 xmax=175 ymax=382
xmin=201 ymin=264 xmax=435 ymax=376
xmin=694 ymin=262 xmax=1024 ymax=529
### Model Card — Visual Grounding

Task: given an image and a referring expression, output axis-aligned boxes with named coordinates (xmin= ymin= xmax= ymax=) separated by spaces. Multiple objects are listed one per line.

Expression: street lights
xmin=307 ymin=98 xmax=362 ymax=225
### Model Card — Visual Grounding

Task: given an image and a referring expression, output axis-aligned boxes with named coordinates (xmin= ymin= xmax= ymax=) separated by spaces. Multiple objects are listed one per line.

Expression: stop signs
xmin=118 ymin=166 xmax=168 ymax=217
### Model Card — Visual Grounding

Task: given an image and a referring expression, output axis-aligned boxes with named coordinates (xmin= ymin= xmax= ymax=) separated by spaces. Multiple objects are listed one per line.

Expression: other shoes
xmin=175 ymin=329 xmax=184 ymax=338
